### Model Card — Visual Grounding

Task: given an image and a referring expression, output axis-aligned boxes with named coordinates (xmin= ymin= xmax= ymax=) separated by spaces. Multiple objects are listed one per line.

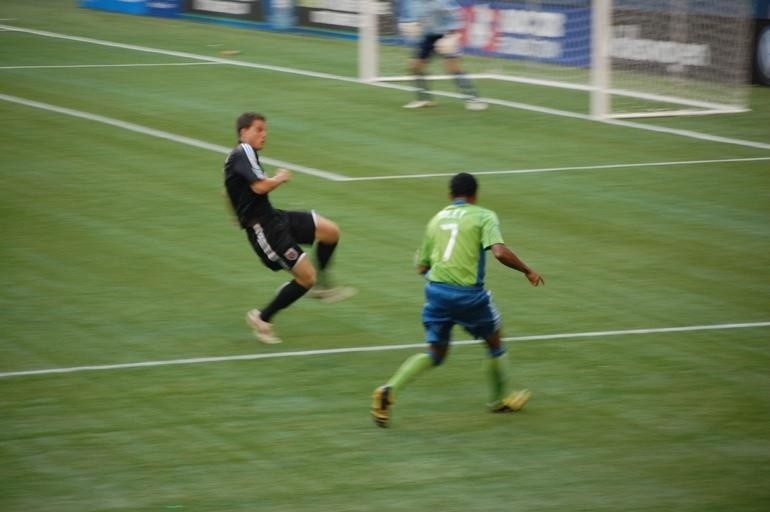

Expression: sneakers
xmin=402 ymin=99 xmax=432 ymax=109
xmin=308 ymin=285 xmax=354 ymax=303
xmin=243 ymin=309 xmax=281 ymax=344
xmin=493 ymin=391 xmax=528 ymax=414
xmin=466 ymin=103 xmax=487 ymax=111
xmin=371 ymin=385 xmax=392 ymax=426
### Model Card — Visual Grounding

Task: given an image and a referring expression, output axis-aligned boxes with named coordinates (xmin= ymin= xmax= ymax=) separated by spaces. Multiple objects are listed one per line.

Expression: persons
xmin=223 ymin=111 xmax=360 ymax=346
xmin=396 ymin=0 xmax=489 ymax=112
xmin=370 ymin=172 xmax=547 ymax=429
